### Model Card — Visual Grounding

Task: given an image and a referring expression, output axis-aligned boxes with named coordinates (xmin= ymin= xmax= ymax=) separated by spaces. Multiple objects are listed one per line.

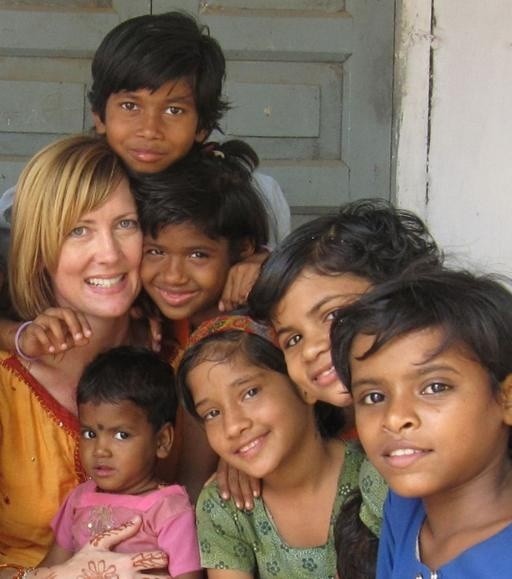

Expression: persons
xmin=0 ymin=10 xmax=293 ymax=361
xmin=247 ymin=196 xmax=446 ymax=579
xmin=131 ymin=139 xmax=276 ymax=344
xmin=172 ymin=309 xmax=369 ymax=579
xmin=329 ymin=252 xmax=511 ymax=578
xmin=0 ymin=133 xmax=190 ymax=578
xmin=31 ymin=346 xmax=205 ymax=579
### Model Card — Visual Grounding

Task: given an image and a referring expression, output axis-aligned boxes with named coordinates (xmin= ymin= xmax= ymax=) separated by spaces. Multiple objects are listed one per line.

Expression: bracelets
xmin=259 ymin=243 xmax=274 ymax=253
xmin=13 ymin=567 xmax=32 ymax=579
xmin=12 ymin=320 xmax=35 ymax=361
